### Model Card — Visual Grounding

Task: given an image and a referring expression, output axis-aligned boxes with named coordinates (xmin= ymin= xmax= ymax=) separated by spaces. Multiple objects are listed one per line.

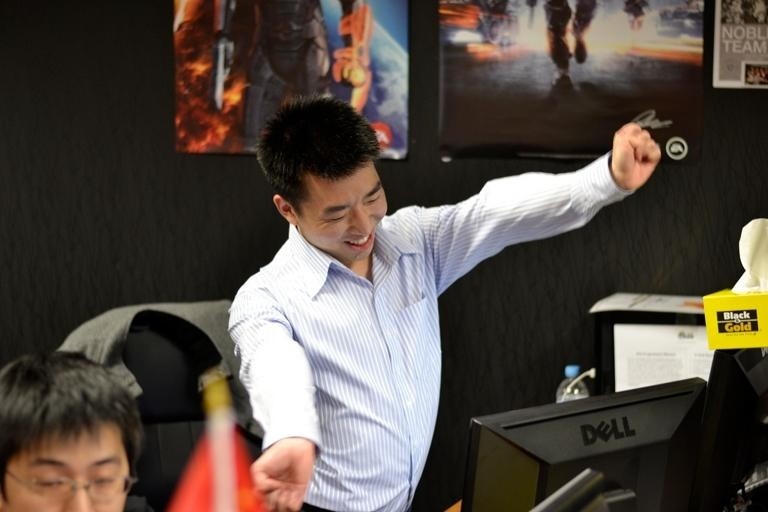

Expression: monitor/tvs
xmin=461 ymin=376 xmax=709 ymax=512
xmin=687 ymin=349 xmax=768 ymax=512
xmin=597 ymin=311 xmax=715 ymax=392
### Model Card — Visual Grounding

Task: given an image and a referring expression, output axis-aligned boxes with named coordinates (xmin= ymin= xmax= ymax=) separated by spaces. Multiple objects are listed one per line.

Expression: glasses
xmin=12 ymin=473 xmax=137 ymax=501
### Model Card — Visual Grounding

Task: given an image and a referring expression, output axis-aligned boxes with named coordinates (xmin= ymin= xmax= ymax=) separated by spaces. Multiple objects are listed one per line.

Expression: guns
xmin=209 ymin=40 xmax=233 ymax=112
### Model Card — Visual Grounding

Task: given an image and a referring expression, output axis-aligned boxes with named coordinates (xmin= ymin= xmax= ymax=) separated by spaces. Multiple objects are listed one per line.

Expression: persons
xmin=1 ymin=348 xmax=147 ymax=512
xmin=226 ymin=95 xmax=662 ymax=512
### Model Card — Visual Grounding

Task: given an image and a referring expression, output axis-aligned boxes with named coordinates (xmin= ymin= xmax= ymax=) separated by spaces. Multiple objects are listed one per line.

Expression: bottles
xmin=556 ymin=365 xmax=590 ymax=405
